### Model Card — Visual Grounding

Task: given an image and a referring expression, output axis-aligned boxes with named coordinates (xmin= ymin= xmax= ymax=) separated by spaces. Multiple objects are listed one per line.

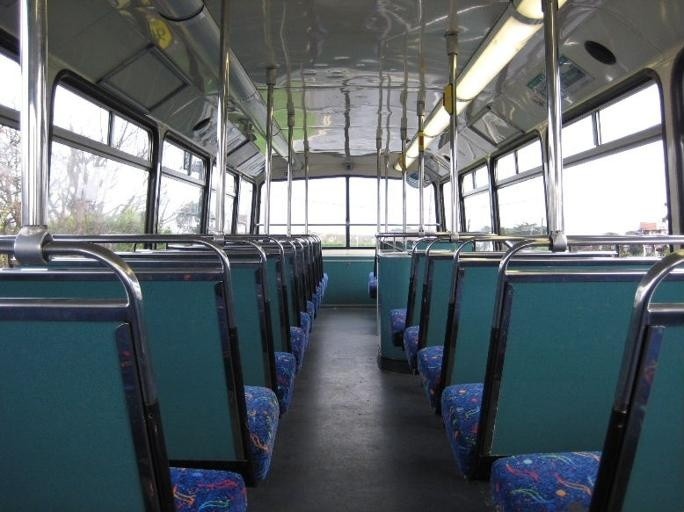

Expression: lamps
xmin=150 ymin=0 xmax=298 ymax=167
xmin=393 ymin=0 xmax=568 ymax=171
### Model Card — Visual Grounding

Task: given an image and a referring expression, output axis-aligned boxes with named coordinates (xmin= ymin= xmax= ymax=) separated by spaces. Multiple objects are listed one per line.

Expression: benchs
xmin=367 ymin=230 xmax=684 ymax=512
xmin=0 ymin=233 xmax=328 ymax=512
